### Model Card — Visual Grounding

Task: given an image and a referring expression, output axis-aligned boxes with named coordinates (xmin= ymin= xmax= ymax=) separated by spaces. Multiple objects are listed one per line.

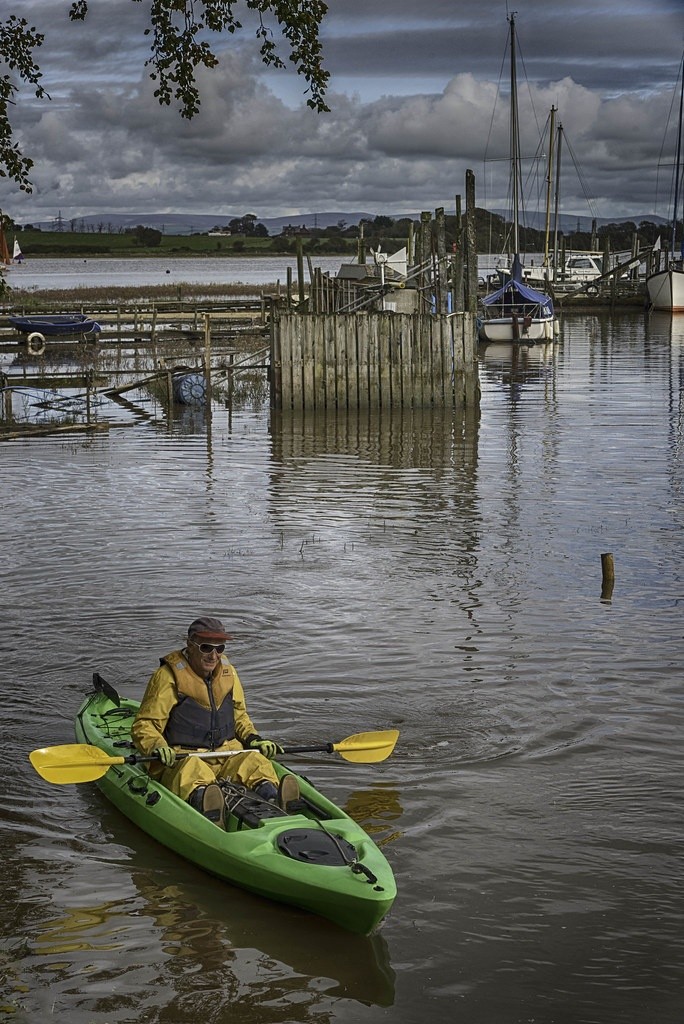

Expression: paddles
xmin=28 ymin=727 xmax=401 ymax=787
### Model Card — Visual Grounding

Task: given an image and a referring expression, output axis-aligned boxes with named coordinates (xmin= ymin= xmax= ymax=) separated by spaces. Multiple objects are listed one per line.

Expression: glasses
xmin=193 ymin=641 xmax=225 ymax=655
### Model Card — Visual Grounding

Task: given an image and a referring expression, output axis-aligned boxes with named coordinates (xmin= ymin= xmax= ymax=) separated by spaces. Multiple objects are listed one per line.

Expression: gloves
xmin=154 ymin=746 xmax=176 ymax=766
xmin=250 ymin=738 xmax=284 ymax=759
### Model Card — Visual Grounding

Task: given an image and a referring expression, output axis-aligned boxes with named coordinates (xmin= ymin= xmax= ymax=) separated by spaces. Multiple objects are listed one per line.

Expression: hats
xmin=188 ymin=617 xmax=233 ymax=640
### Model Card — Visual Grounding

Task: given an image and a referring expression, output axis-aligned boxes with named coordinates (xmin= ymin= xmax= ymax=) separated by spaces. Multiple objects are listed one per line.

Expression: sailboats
xmin=12 ymin=234 xmax=26 ymax=265
xmin=476 ymin=13 xmax=684 ymax=340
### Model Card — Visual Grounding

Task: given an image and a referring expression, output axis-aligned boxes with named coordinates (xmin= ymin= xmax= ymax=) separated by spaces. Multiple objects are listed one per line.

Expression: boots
xmin=189 ymin=783 xmax=225 ymax=830
xmin=252 ymin=774 xmax=299 ymax=815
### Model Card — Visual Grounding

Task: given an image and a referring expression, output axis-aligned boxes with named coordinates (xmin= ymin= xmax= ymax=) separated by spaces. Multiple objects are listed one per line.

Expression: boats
xmin=76 ymin=671 xmax=395 ymax=934
xmin=7 ymin=312 xmax=102 ymax=336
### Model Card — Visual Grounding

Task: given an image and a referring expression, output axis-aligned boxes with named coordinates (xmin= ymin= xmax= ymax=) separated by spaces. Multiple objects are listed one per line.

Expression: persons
xmin=130 ymin=616 xmax=299 ymax=830
